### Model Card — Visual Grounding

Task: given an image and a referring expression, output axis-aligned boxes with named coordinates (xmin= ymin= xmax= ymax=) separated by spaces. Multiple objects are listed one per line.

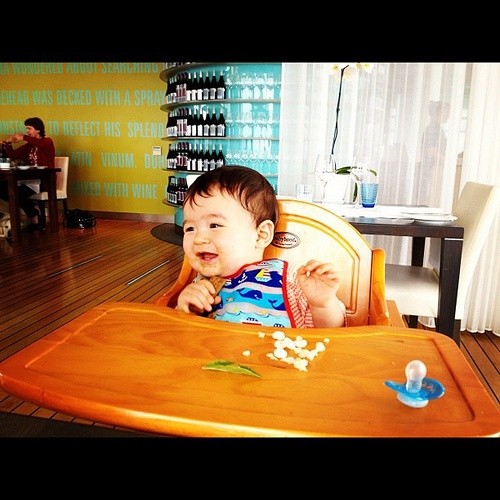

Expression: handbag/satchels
xmin=65 ymin=208 xmax=96 ymax=228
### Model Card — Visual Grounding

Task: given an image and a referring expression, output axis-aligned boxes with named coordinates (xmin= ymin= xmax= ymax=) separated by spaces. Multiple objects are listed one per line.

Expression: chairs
xmin=384 ymin=181 xmax=500 ymax=349
xmin=0 ymin=193 xmax=500 ymax=440
xmin=29 ymin=156 xmax=69 ymax=233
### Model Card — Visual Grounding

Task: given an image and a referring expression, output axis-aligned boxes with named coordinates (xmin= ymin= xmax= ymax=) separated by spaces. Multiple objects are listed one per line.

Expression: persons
xmin=174 ymin=165 xmax=347 ymax=330
xmin=3 ymin=117 xmax=55 ymax=230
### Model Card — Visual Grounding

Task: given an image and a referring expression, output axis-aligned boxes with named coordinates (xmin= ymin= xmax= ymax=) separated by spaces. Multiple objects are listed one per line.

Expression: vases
xmin=319 ymin=175 xmax=357 ymax=202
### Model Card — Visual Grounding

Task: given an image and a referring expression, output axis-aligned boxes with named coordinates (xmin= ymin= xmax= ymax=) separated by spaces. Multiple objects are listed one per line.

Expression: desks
xmin=0 ymin=167 xmax=62 ymax=241
xmin=327 ymin=203 xmax=464 ymax=339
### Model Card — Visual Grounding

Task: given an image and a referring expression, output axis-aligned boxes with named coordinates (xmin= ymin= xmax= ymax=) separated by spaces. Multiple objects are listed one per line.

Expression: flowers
xmin=323 ymin=62 xmax=377 ymax=204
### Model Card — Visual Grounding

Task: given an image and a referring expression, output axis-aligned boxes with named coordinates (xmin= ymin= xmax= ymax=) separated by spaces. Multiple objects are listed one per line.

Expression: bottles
xmin=167 ymin=61 xmax=226 ymax=206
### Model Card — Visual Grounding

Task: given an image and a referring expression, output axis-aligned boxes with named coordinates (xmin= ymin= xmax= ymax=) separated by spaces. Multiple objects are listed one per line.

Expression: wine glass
xmin=350 ymin=154 xmax=369 ymax=210
xmin=314 ymin=153 xmax=337 ymax=203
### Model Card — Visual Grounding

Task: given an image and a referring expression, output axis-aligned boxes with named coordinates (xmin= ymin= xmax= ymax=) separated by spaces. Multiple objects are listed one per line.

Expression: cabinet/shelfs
xmin=150 ymin=63 xmax=282 ymax=246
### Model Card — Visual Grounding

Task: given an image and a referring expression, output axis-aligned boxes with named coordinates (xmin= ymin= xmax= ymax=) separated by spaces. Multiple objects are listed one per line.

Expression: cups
xmin=361 ymin=176 xmax=379 ymax=208
xmin=296 ymin=184 xmax=316 ymax=204
xmin=0 ymin=162 xmax=11 ymax=168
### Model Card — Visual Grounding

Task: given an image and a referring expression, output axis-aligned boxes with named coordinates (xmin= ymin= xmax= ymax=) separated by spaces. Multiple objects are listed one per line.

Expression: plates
xmin=16 ymin=166 xmax=30 ymax=170
xmin=34 ymin=166 xmax=49 ymax=170
xmin=325 ymin=207 xmax=459 ymax=225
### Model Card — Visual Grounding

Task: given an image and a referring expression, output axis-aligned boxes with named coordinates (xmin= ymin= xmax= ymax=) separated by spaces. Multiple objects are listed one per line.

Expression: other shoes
xmin=32 ymin=209 xmax=39 ymax=228
